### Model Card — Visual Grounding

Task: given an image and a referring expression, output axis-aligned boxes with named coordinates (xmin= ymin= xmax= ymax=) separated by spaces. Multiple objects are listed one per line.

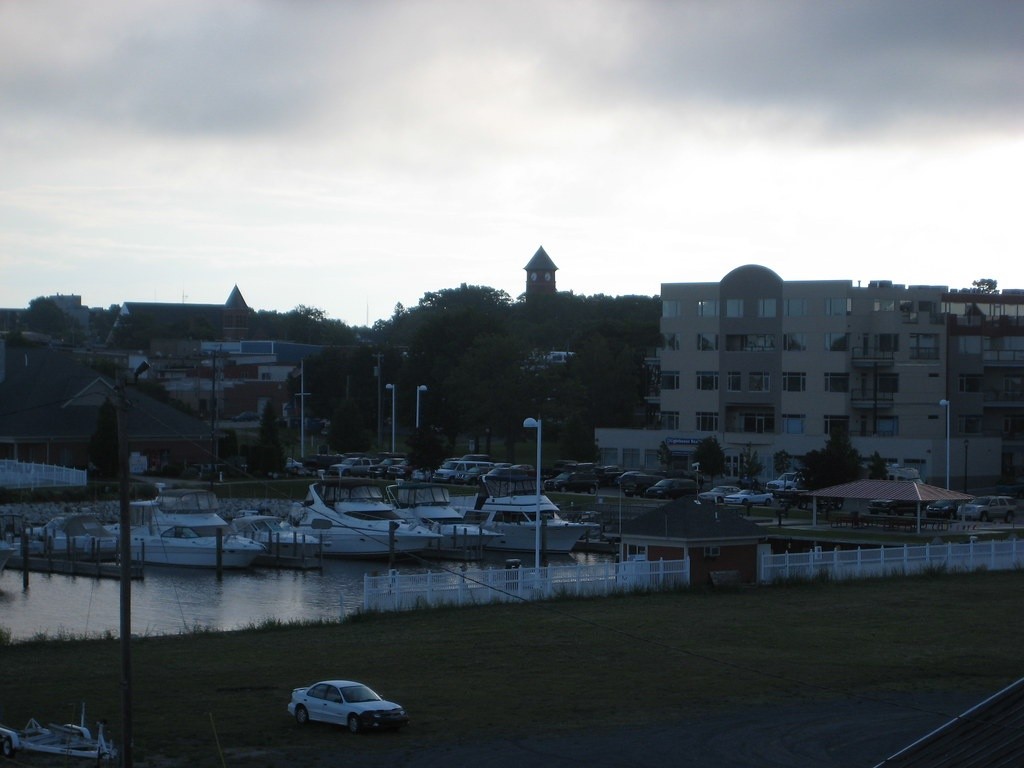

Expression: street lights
xmin=521 ymin=418 xmax=543 ymax=600
xmin=385 ymin=382 xmax=398 ymax=456
xmin=940 ymin=400 xmax=952 ymax=495
xmin=414 ymin=385 xmax=428 ymax=431
xmin=962 ymin=436 xmax=970 ymax=494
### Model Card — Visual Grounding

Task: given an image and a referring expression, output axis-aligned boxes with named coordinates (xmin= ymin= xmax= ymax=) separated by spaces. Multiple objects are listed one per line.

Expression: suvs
xmin=868 ymin=499 xmax=923 ymax=517
xmin=765 ymin=472 xmax=806 ymax=498
xmin=957 ymin=495 xmax=1018 ymax=524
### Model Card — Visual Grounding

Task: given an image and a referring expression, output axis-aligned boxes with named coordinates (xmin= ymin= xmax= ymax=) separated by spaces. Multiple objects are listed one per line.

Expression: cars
xmin=285 ymin=458 xmax=304 ymax=473
xmin=302 ymin=451 xmax=704 ymax=500
xmin=723 ymin=488 xmax=775 ymax=506
xmin=287 ymin=679 xmax=410 ymax=735
xmin=698 ymin=485 xmax=742 ymax=504
xmin=925 ymin=500 xmax=963 ymax=520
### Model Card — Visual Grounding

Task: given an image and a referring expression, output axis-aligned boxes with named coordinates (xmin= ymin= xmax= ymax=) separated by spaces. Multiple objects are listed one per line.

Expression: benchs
xmin=829 ymin=515 xmax=960 ymax=532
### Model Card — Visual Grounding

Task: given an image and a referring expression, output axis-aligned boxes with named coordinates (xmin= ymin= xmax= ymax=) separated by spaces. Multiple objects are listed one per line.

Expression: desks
xmin=829 ymin=515 xmax=960 ymax=531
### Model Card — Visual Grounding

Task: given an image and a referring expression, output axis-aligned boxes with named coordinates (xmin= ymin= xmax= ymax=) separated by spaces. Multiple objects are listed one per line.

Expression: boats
xmin=223 ymin=502 xmax=320 ymax=557
xmin=451 ymin=465 xmax=603 ymax=554
xmin=0 ymin=512 xmax=52 ymax=557
xmin=385 ymin=478 xmax=508 ymax=546
xmin=25 ymin=510 xmax=118 ymax=551
xmin=102 ymin=488 xmax=268 ymax=568
xmin=284 ymin=467 xmax=444 ymax=556
